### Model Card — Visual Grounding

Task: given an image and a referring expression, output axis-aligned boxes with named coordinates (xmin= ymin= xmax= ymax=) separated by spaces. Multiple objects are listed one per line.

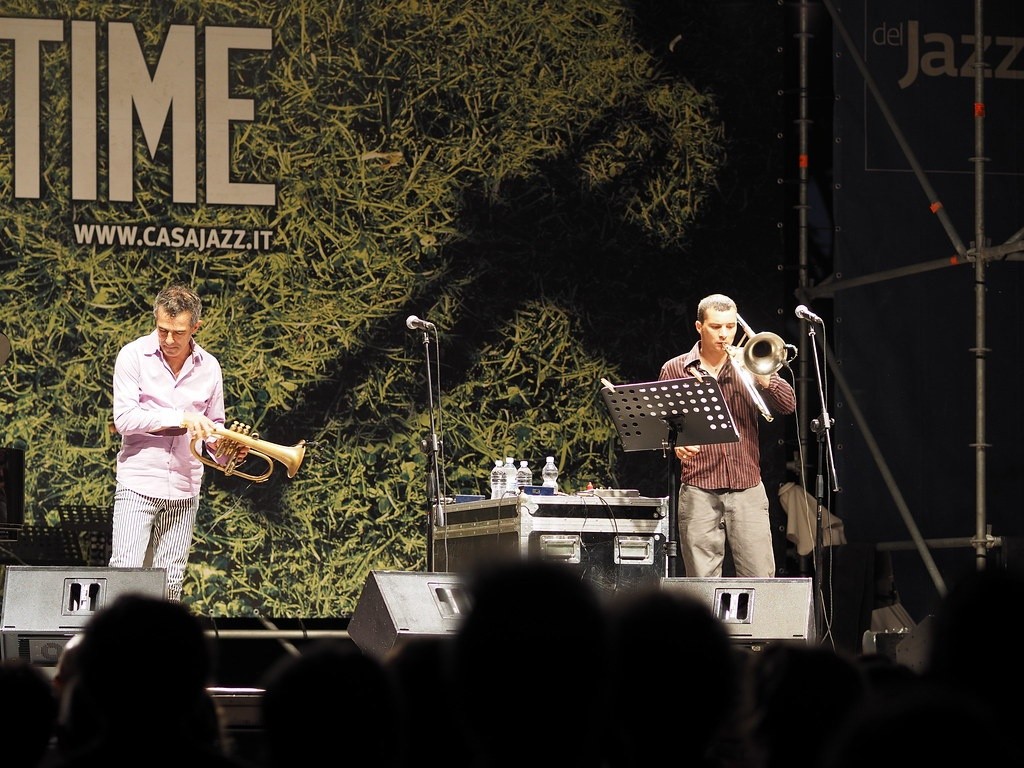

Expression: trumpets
xmin=177 ymin=419 xmax=306 ymax=484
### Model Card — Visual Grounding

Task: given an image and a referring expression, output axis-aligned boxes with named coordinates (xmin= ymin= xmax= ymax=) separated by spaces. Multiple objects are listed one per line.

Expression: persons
xmin=0 ymin=544 xmax=1024 ymax=768
xmin=105 ymin=283 xmax=250 ymax=605
xmin=655 ymin=293 xmax=797 ymax=580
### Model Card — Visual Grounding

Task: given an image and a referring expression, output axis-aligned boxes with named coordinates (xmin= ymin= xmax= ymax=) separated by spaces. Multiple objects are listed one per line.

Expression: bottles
xmin=542 ymin=456 xmax=558 ymax=495
xmin=491 ymin=460 xmax=506 ymax=500
xmin=503 ymin=457 xmax=519 ymax=497
xmin=517 ymin=460 xmax=532 ymax=487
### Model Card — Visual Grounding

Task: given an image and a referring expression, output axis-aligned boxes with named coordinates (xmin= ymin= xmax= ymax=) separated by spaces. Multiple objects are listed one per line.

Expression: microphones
xmin=406 ymin=317 xmax=439 ymax=331
xmin=796 ymin=303 xmax=826 ymax=328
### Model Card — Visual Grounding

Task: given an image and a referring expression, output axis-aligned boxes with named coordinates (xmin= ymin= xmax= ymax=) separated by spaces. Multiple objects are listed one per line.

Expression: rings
xmin=683 ymin=453 xmax=688 ymax=459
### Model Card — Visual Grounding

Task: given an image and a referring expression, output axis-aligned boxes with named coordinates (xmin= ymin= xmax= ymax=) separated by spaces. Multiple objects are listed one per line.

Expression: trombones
xmin=724 ymin=313 xmax=788 ymax=423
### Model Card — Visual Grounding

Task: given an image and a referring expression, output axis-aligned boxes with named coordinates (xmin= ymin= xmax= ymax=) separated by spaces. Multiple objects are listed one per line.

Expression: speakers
xmin=0 ymin=566 xmax=169 ymax=671
xmin=345 ymin=568 xmax=478 ymax=664
xmin=658 ymin=577 xmax=817 ymax=653
